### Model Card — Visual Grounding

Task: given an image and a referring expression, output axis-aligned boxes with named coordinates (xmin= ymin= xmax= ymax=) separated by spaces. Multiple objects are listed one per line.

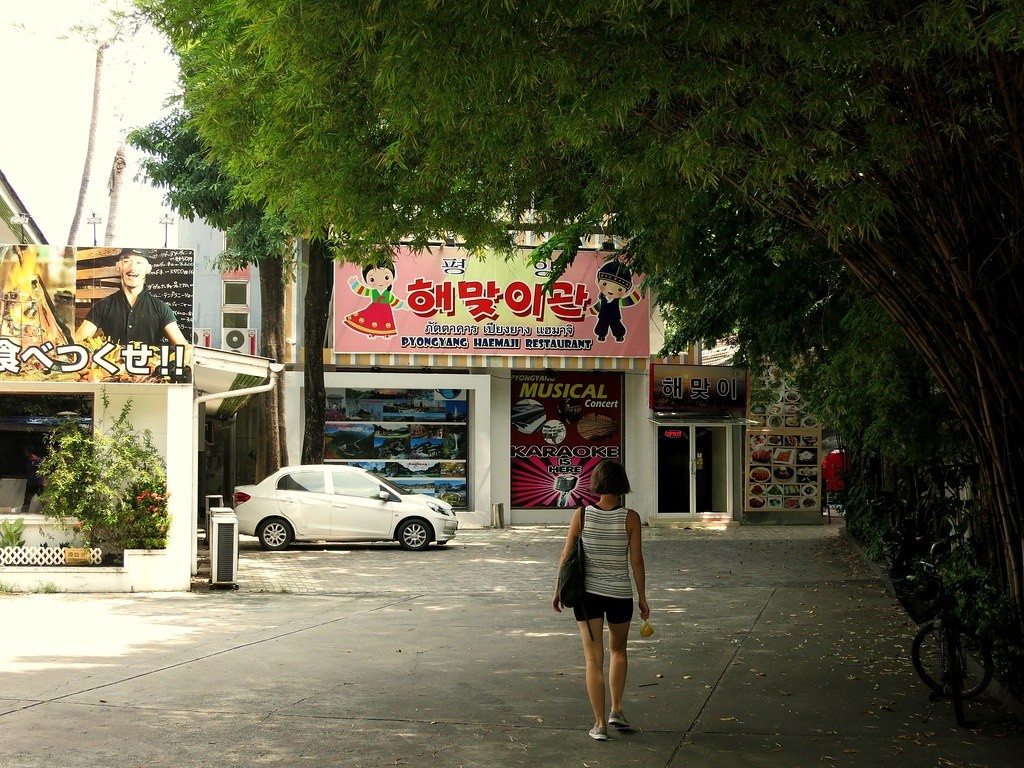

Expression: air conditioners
xmin=205 ymin=419 xmax=214 ymax=445
xmin=193 ymin=328 xmax=211 ymax=347
xmin=206 ymin=495 xmax=224 ymax=543
xmin=210 ymin=519 xmax=239 ymax=590
xmin=209 ymin=508 xmax=234 ymax=517
xmin=221 ymin=328 xmax=257 ymax=355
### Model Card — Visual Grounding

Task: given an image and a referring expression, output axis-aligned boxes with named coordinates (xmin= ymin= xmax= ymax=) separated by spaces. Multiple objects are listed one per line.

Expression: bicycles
xmin=890 ymin=533 xmax=995 ymax=728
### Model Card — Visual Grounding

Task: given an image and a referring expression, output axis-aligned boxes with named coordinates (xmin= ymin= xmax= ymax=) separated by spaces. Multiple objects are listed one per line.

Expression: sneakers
xmin=587 ymin=725 xmax=608 ymax=740
xmin=608 ymin=711 xmax=631 ymax=727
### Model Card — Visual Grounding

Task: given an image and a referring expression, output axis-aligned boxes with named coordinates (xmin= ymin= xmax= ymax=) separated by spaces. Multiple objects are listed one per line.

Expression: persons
xmin=552 ymin=459 xmax=648 ymax=740
xmin=75 ymin=248 xmax=187 ymax=346
xmin=20 ymin=447 xmax=46 ymax=513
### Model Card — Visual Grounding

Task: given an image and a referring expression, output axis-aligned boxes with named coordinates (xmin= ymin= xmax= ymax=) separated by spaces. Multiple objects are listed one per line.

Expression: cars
xmin=234 ymin=464 xmax=459 ymax=550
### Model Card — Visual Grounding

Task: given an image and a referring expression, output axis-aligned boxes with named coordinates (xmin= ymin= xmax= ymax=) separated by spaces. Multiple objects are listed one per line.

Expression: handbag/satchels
xmin=559 ymin=503 xmax=588 ymax=608
